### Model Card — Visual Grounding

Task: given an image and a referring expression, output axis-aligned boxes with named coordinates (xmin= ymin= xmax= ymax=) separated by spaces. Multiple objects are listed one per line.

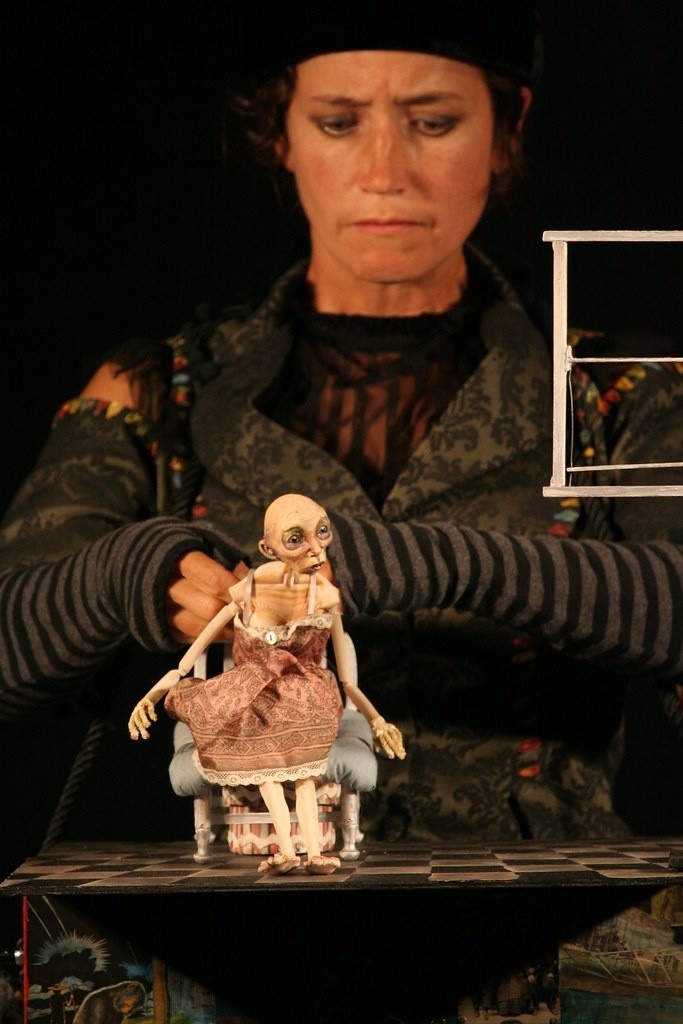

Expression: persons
xmin=128 ymin=493 xmax=408 ymax=872
xmin=0 ymin=0 xmax=683 ymax=1024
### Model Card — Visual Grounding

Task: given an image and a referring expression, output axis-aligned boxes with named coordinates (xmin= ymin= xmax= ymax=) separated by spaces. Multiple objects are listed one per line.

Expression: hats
xmin=269 ymin=0 xmax=544 ymax=90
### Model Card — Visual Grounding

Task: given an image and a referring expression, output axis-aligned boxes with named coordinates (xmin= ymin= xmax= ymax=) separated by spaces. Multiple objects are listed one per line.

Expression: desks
xmin=0 ymin=838 xmax=683 ymax=1024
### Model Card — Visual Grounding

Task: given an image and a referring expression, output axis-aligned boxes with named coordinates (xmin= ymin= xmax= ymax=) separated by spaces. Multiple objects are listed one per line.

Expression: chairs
xmin=169 ymin=631 xmax=377 ymax=862
